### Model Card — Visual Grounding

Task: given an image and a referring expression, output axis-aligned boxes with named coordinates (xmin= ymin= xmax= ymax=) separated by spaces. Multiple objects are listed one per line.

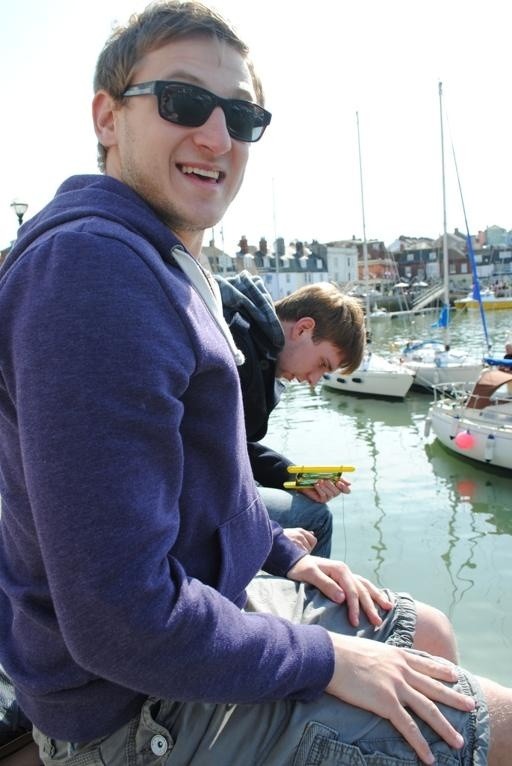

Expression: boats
xmin=453 ymin=288 xmax=512 ymax=312
xmin=424 ymin=357 xmax=512 ymax=470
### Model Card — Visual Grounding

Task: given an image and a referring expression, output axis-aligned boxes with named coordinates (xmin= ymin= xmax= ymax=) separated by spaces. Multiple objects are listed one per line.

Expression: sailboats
xmin=318 ymin=107 xmax=416 ymax=398
xmin=391 ymin=81 xmax=484 ymax=393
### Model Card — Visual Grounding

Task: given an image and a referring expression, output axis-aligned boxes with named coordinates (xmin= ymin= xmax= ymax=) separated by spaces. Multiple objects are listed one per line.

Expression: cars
xmin=347 ymin=280 xmax=414 ymax=313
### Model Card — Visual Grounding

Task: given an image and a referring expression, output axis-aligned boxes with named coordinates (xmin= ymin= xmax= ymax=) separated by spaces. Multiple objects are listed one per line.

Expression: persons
xmin=294 ymin=241 xmax=304 ymax=268
xmin=0 ymin=1 xmax=512 ymax=765
xmin=236 ymin=235 xmax=249 ymax=257
xmin=404 ymin=344 xmax=411 ymax=353
xmin=255 ymin=236 xmax=266 ymax=267
xmin=214 ymin=269 xmax=366 ymax=557
xmin=503 ymin=343 xmax=512 ymax=359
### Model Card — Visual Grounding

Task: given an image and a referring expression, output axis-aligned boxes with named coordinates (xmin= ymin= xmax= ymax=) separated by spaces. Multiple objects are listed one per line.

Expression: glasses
xmin=121 ymin=79 xmax=273 ymax=144
